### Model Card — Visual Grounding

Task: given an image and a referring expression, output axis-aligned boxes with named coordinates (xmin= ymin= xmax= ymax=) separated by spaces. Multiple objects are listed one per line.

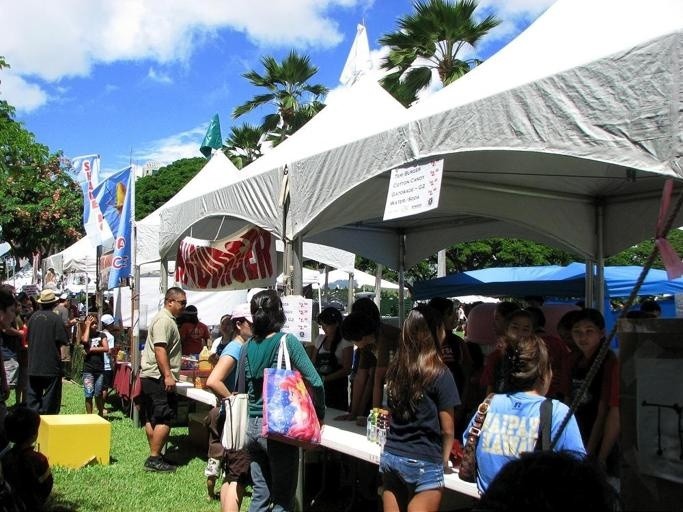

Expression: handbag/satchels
xmin=459 ymin=434 xmax=478 ymax=482
xmin=230 ymin=302 xmax=253 ymax=323
xmin=221 ymin=347 xmax=321 ymax=451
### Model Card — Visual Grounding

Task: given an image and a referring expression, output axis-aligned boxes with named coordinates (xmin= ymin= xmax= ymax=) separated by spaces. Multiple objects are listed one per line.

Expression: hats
xmin=36 ymin=289 xmax=59 ymax=304
xmin=100 ymin=314 xmax=114 ymax=325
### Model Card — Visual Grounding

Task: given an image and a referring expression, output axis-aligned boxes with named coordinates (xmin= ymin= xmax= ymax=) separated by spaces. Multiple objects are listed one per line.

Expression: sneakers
xmin=144 ymin=454 xmax=177 ymax=472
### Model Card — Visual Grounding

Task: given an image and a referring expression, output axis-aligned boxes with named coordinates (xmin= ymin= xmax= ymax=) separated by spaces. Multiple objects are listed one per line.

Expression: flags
xmin=68 ymin=152 xmax=96 ymax=235
xmin=89 ymin=164 xmax=130 ymax=289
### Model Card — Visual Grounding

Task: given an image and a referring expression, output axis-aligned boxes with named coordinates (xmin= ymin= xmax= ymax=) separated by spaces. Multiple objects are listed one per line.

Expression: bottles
xmin=198 ymin=345 xmax=209 ymax=360
xmin=365 ymin=407 xmax=389 ymax=449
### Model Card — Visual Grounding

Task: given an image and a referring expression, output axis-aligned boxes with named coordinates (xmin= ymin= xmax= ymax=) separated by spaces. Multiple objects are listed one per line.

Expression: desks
xmin=157 ymin=369 xmax=622 ymax=507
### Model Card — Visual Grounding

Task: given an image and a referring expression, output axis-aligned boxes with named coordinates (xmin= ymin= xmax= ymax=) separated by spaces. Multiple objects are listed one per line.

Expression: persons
xmin=624 ymin=300 xmax=660 ymax=318
xmin=180 ymin=306 xmax=209 ymax=355
xmin=0 ymin=287 xmax=77 ymax=416
xmin=203 ymin=289 xmax=618 ymax=512
xmin=140 ymin=288 xmax=187 ymax=471
xmin=0 ymin=409 xmax=52 ymax=512
xmin=78 ymin=295 xmax=122 ymax=417
